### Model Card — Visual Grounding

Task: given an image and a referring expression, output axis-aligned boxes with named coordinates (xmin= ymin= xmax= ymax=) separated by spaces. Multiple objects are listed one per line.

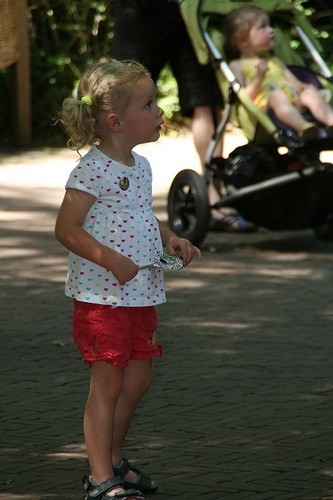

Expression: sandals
xmin=112 ymin=457 xmax=160 ymax=495
xmin=82 ymin=472 xmax=145 ymax=500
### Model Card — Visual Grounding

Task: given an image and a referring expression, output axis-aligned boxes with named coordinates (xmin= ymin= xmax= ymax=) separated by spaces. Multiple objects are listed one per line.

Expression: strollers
xmin=165 ymin=0 xmax=333 ymax=250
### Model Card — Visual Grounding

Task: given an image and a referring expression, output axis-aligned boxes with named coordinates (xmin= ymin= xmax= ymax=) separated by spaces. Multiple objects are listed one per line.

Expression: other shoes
xmin=210 ymin=211 xmax=256 ymax=233
xmin=299 ymin=121 xmax=333 ymax=152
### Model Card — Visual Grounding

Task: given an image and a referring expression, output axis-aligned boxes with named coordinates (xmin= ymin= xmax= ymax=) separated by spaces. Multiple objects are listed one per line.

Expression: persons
xmin=223 ymin=6 xmax=333 ymax=141
xmin=54 ymin=60 xmax=202 ymax=500
xmin=104 ymin=0 xmax=258 ymax=232
